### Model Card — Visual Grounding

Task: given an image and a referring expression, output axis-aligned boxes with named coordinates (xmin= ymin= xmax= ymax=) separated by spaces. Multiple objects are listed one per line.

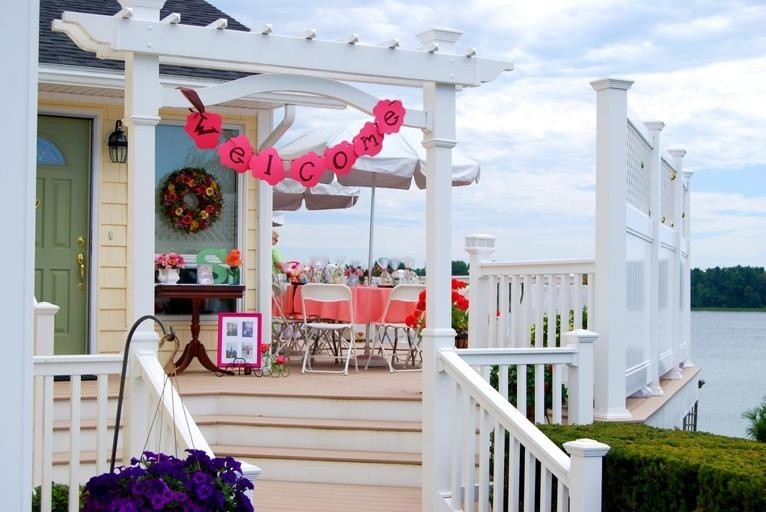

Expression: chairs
xmin=270 ymin=261 xmax=426 ymax=375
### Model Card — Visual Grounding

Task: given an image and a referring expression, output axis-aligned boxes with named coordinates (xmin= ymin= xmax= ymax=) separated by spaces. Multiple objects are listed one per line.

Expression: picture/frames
xmin=217 ymin=312 xmax=262 ymax=368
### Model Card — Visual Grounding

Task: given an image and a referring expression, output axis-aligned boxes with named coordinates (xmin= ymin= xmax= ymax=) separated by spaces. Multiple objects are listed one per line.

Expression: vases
xmin=157 ymin=268 xmax=181 ymax=285
xmin=227 ymin=265 xmax=240 ymax=285
xmin=454 ymin=333 xmax=470 ymax=349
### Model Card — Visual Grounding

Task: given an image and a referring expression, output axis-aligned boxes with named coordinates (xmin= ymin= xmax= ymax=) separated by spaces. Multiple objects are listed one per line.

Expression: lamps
xmin=107 ymin=120 xmax=127 ymax=163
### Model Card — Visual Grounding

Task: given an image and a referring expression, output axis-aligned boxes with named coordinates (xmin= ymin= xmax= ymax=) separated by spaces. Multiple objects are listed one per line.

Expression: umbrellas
xmin=273 ymin=174 xmax=361 ymax=211
xmin=268 ymin=119 xmax=480 ymax=358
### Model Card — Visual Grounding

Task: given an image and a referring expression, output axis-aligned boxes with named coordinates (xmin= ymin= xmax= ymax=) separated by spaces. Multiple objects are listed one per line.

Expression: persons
xmin=273 ymin=228 xmax=285 ymax=281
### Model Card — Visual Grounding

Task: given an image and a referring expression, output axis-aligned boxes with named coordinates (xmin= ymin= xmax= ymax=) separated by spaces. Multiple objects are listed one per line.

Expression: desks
xmin=153 ymin=281 xmax=246 ymax=377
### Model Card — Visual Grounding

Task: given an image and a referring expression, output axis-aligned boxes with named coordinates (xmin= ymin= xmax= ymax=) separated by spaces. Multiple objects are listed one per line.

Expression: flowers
xmin=403 ymin=274 xmax=501 ymax=335
xmin=153 ymin=251 xmax=186 ymax=270
xmin=274 ymin=354 xmax=289 ymax=364
xmin=259 ymin=342 xmax=270 ymax=355
xmin=79 ymin=446 xmax=256 ymax=512
xmin=227 ymin=248 xmax=245 ymax=268
xmin=158 ymin=166 xmax=224 ymax=234
